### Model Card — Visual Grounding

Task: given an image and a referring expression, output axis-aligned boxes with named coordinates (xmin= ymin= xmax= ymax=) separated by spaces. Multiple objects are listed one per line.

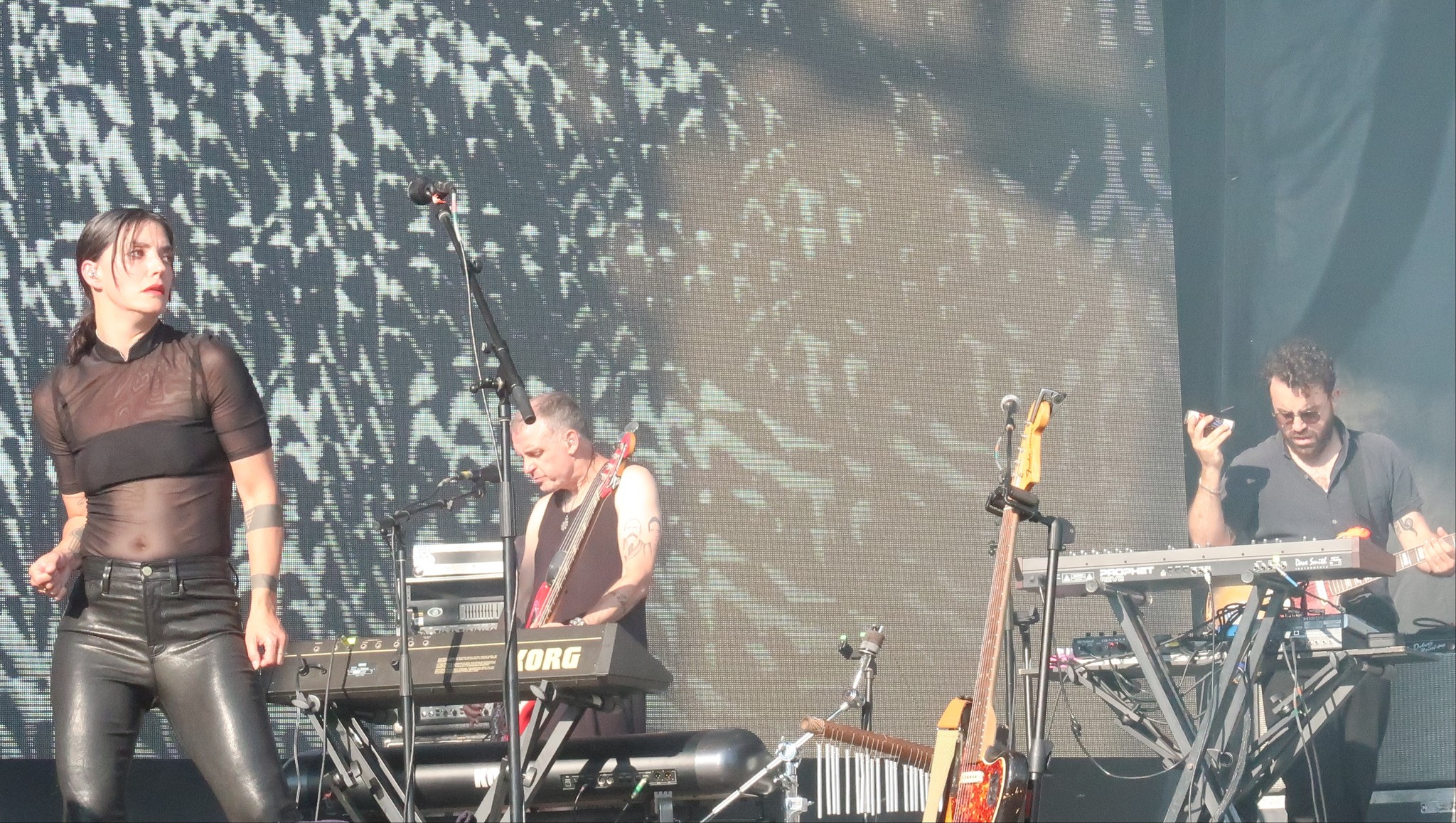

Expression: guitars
xmin=481 ymin=432 xmax=635 ymax=746
xmin=938 ymin=386 xmax=1069 ymax=823
xmin=1205 ymin=531 xmax=1456 ymax=634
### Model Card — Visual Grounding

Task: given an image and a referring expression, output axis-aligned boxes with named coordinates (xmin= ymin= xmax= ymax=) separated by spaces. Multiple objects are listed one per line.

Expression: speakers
xmin=1037 ymin=757 xmax=1189 ymax=823
xmin=1360 ymin=639 xmax=1456 ymax=789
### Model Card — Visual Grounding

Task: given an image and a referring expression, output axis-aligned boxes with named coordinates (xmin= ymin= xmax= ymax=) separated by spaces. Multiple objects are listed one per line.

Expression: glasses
xmin=1270 ymin=394 xmax=1329 ymax=425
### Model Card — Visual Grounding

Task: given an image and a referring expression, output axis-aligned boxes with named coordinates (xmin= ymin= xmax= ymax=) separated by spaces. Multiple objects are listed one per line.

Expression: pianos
xmin=265 ymin=622 xmax=674 ymax=823
xmin=1015 ymin=538 xmax=1456 ymax=823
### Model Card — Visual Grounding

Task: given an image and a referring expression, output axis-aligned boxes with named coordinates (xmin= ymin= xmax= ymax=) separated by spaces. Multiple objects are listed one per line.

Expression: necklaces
xmin=560 ymin=447 xmax=594 ymax=531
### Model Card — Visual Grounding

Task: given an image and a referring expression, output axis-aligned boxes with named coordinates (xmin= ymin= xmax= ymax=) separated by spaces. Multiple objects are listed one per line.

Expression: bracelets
xmin=569 ymin=616 xmax=587 ymax=626
xmin=1199 ymin=477 xmax=1223 ymax=495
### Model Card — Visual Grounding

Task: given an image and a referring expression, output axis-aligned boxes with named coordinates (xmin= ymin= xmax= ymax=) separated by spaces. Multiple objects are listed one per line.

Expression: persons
xmin=462 ymin=392 xmax=662 ymax=739
xmin=28 ymin=207 xmax=305 ymax=823
xmin=1187 ymin=339 xmax=1456 ymax=823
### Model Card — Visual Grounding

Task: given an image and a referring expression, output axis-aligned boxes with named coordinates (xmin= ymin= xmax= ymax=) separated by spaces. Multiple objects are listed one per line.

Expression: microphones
xmin=453 ymin=459 xmax=506 ymax=485
xmin=408 ymin=178 xmax=454 ymax=204
xmin=1000 ymin=394 xmax=1021 ymax=420
